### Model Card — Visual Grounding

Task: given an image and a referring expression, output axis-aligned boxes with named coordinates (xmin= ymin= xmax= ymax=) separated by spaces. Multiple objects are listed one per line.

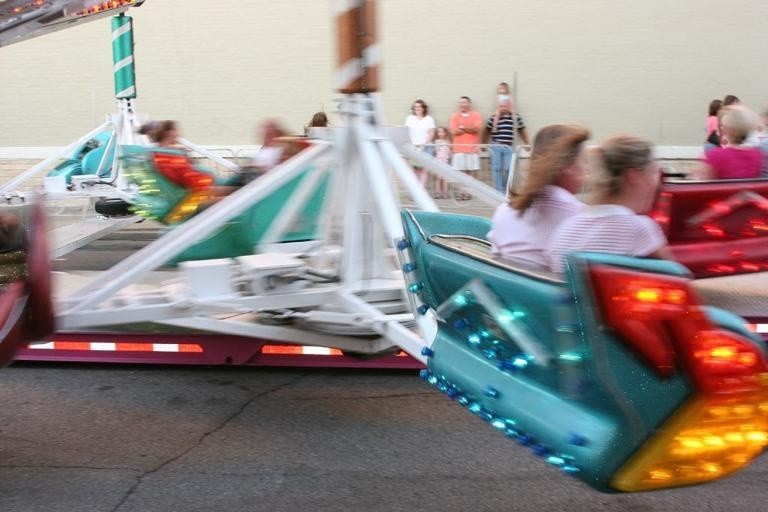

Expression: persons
xmin=404 ymin=99 xmax=437 ymax=201
xmin=484 ymin=82 xmax=532 ymax=195
xmin=486 ymin=124 xmax=592 ymax=274
xmin=303 ymin=111 xmax=336 ymax=128
xmin=140 ymin=118 xmax=215 ymax=190
xmin=432 ymin=127 xmax=452 ymax=203
xmin=545 ymin=133 xmax=678 ymax=276
xmin=686 ymin=94 xmax=768 ymax=180
xmin=449 ymin=96 xmax=483 ymax=201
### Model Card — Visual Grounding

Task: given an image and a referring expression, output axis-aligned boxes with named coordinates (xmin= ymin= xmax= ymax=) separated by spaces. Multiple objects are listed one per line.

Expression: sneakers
xmin=433 ymin=192 xmax=471 ymax=200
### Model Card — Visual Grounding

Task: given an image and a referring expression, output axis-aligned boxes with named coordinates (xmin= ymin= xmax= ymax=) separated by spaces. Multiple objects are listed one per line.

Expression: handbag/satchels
xmin=707 ymin=130 xmax=720 ymax=146
